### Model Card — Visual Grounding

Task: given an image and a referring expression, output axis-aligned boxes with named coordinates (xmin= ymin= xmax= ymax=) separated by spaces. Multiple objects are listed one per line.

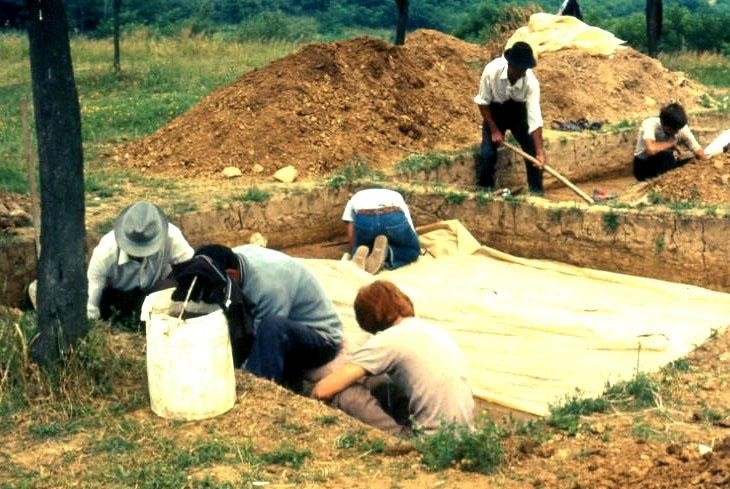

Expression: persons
xmin=339 ymin=188 xmax=422 ymax=274
xmin=87 ymin=199 xmax=196 ymax=322
xmin=633 ymin=101 xmax=709 ymax=184
xmin=307 ymin=277 xmax=477 ymax=444
xmin=188 ymin=241 xmax=345 ymax=397
xmin=474 ymin=41 xmax=547 ymax=197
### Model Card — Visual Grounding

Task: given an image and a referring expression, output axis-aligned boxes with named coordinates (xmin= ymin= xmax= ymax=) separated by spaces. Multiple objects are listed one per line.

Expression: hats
xmin=114 ymin=202 xmax=168 ymax=257
xmin=505 ymin=42 xmax=536 ymax=68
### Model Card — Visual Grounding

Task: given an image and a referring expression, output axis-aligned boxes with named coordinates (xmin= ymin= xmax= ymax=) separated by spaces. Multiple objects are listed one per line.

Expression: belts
xmin=358 ymin=207 xmax=399 ymax=214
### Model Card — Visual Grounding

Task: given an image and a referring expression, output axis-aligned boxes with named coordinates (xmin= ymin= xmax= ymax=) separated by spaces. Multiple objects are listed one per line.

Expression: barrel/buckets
xmin=144 ymin=276 xmax=237 ymax=423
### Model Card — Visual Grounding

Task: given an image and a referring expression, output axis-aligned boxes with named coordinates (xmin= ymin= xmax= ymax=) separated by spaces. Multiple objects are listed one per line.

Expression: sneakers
xmin=352 ymin=234 xmax=387 ymax=274
